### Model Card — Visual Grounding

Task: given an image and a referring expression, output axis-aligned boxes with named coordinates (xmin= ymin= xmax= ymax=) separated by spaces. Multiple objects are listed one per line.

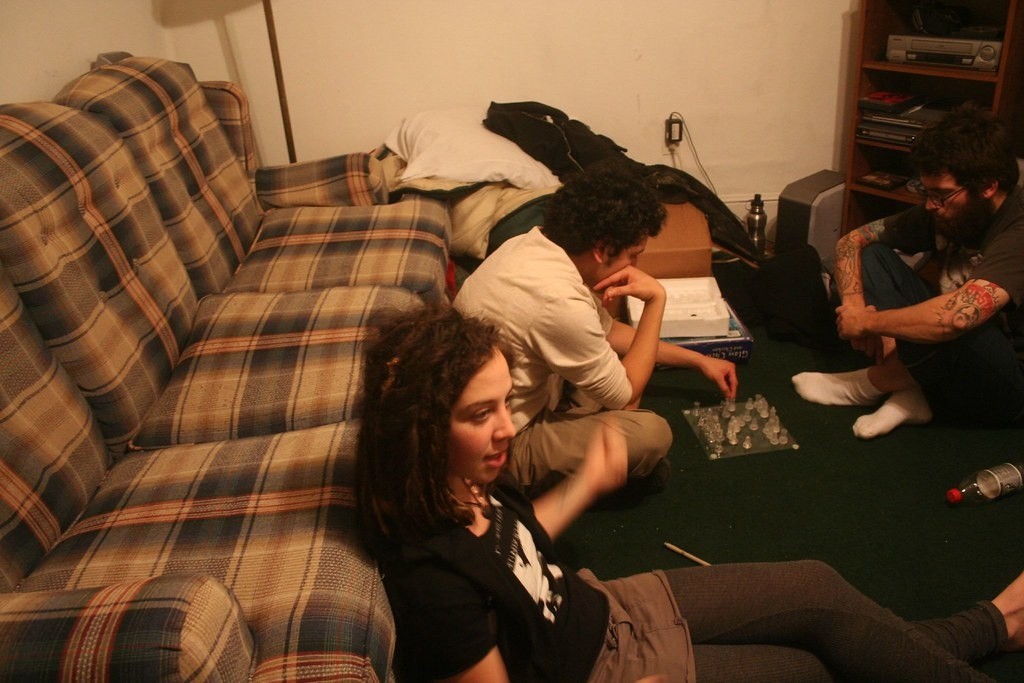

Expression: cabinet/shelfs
xmin=839 ymin=0 xmax=1023 ymax=238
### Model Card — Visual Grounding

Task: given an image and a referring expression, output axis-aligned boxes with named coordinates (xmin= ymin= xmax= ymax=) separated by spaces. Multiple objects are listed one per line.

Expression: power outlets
xmin=661 ymin=125 xmax=682 ymax=154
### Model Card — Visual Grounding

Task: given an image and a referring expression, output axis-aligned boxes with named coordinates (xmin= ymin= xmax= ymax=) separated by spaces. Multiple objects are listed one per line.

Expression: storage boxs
xmin=634 ymin=199 xmax=713 ymax=279
xmin=626 ymin=301 xmax=754 ymax=371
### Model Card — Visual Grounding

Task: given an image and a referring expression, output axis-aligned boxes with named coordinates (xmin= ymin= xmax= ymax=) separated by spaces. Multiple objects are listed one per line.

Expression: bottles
xmin=745 ymin=194 xmax=767 ymax=253
xmin=946 ymin=458 xmax=1024 ymax=503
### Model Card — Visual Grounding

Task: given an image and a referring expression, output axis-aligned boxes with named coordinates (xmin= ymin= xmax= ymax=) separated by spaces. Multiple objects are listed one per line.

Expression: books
xmin=855 ymin=90 xmax=948 ymax=153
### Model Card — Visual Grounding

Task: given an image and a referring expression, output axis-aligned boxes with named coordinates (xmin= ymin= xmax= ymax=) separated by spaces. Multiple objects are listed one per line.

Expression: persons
xmin=355 ymin=305 xmax=1024 ymax=683
xmin=791 ymin=105 xmax=1024 ymax=438
xmin=449 ymin=168 xmax=739 ymax=499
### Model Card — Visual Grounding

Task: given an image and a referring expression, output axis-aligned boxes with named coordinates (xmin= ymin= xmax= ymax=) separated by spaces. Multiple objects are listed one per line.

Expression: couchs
xmin=0 ymin=51 xmax=454 ymax=683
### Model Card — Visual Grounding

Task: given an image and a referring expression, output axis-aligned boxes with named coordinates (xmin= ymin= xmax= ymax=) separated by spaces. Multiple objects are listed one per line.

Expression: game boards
xmin=682 ymin=393 xmax=799 ymax=459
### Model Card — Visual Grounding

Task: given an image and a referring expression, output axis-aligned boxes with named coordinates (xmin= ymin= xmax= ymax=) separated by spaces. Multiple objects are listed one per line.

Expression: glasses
xmin=915 ymin=182 xmax=966 ymax=207
